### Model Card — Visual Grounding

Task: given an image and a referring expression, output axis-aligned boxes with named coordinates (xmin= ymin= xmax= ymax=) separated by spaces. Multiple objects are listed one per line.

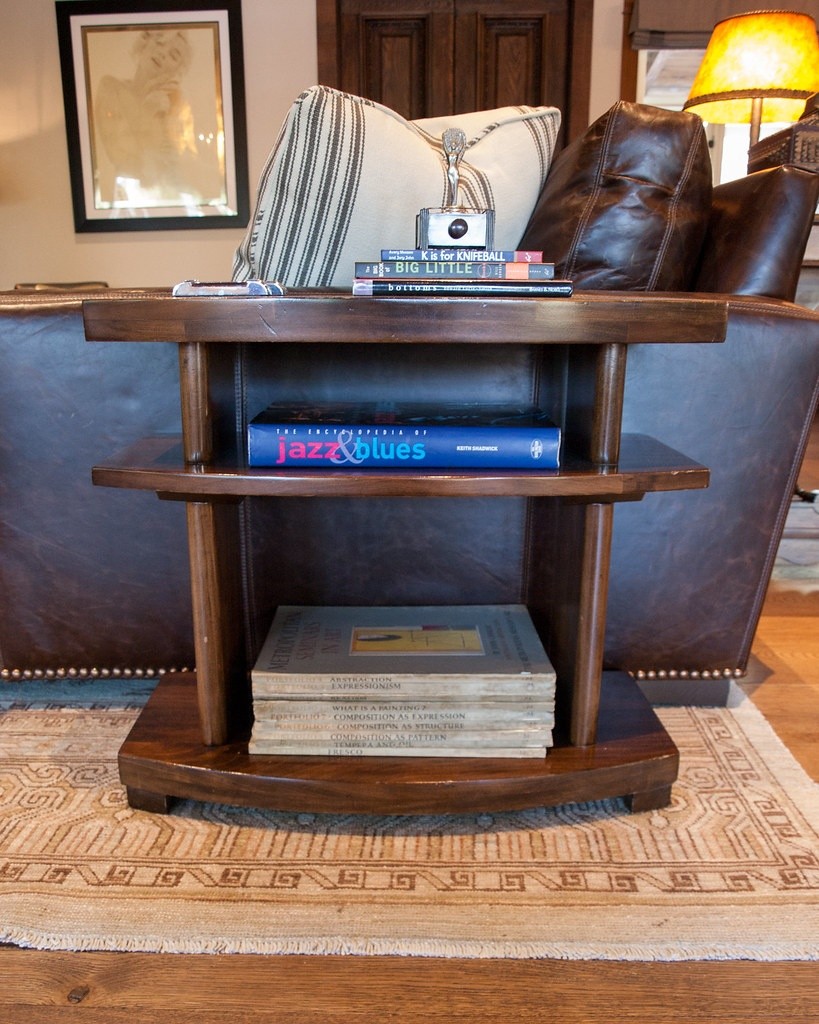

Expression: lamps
xmin=683 ymin=12 xmax=817 ymax=502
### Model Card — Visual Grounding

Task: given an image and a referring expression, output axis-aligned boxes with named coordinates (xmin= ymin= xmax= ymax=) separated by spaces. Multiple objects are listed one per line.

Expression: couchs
xmin=3 ymin=99 xmax=819 ymax=709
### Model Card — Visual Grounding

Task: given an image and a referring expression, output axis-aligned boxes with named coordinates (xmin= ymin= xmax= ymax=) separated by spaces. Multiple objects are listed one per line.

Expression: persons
xmin=442 ymin=135 xmax=464 ymax=206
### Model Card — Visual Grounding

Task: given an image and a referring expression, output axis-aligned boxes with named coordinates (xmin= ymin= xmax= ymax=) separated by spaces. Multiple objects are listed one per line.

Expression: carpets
xmin=0 ymin=666 xmax=818 ymax=959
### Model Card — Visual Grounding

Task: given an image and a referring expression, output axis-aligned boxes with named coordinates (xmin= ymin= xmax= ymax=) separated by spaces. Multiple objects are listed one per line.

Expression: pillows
xmin=225 ymin=86 xmax=564 ymax=283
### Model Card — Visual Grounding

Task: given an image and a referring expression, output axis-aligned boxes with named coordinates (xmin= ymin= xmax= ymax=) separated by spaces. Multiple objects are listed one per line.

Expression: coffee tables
xmin=76 ymin=284 xmax=734 ymax=811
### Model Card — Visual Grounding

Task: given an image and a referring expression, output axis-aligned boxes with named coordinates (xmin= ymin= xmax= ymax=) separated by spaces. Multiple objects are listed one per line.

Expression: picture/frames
xmin=55 ymin=1 xmax=251 ymax=232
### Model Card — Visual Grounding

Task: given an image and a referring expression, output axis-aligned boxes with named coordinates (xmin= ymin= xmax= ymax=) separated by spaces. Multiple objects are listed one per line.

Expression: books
xmin=249 ymin=604 xmax=558 ymax=758
xmin=352 ymin=246 xmax=574 ymax=297
xmin=247 ymin=398 xmax=562 ymax=470
